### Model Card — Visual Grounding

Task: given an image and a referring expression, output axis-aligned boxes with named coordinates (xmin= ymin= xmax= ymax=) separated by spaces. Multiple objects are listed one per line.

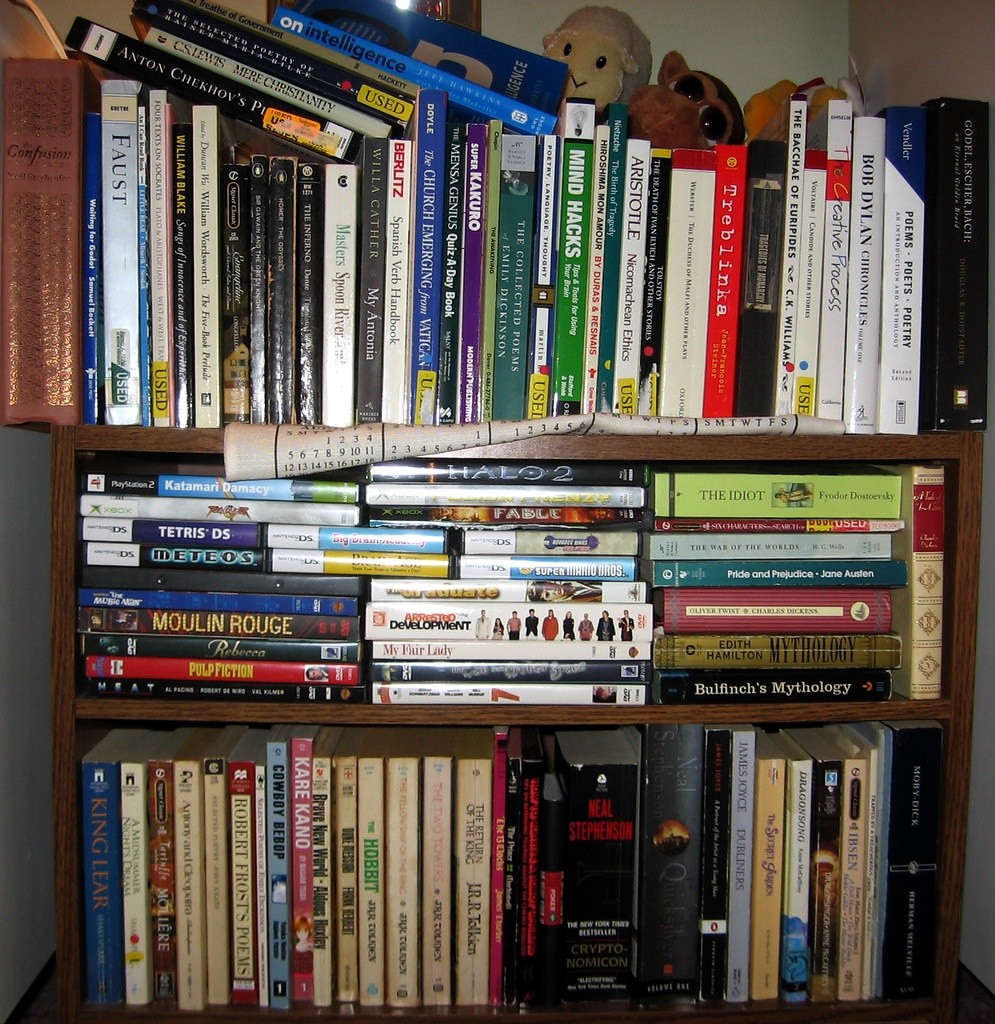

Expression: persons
xmin=493 ymin=618 xmax=504 ymax=639
xmin=577 ymin=614 xmax=594 ymax=641
xmin=619 ymin=610 xmax=635 ymax=641
xmin=474 ymin=610 xmax=491 ymax=639
xmin=596 ymin=611 xmax=616 ymax=641
xmin=596 ymin=688 xmax=616 ymax=703
xmin=506 ymin=611 xmax=521 ymax=640
xmin=525 ymin=609 xmax=539 ymax=640
xmin=526 ymin=581 xmax=603 ymax=601
xmin=306 ymin=666 xmax=328 ymax=680
xmin=541 ymin=609 xmax=559 ymax=641
xmin=563 ymin=611 xmax=575 ymax=641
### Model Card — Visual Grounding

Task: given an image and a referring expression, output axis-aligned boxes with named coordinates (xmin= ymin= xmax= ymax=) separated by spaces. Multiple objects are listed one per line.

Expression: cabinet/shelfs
xmin=53 ymin=424 xmax=986 ymax=1024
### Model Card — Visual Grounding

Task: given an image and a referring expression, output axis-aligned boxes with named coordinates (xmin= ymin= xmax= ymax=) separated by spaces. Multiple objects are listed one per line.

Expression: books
xmin=0 ymin=0 xmax=989 ymax=436
xmin=85 ymin=720 xmax=943 ymax=1007
xmin=72 ymin=455 xmax=942 ymax=707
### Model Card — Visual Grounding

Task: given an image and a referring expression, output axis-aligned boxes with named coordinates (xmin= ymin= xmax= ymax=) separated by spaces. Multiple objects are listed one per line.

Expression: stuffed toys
xmin=743 ymin=77 xmax=866 ymax=144
xmin=628 ymin=50 xmax=745 ymax=151
xmin=541 ymin=6 xmax=651 ymax=116
